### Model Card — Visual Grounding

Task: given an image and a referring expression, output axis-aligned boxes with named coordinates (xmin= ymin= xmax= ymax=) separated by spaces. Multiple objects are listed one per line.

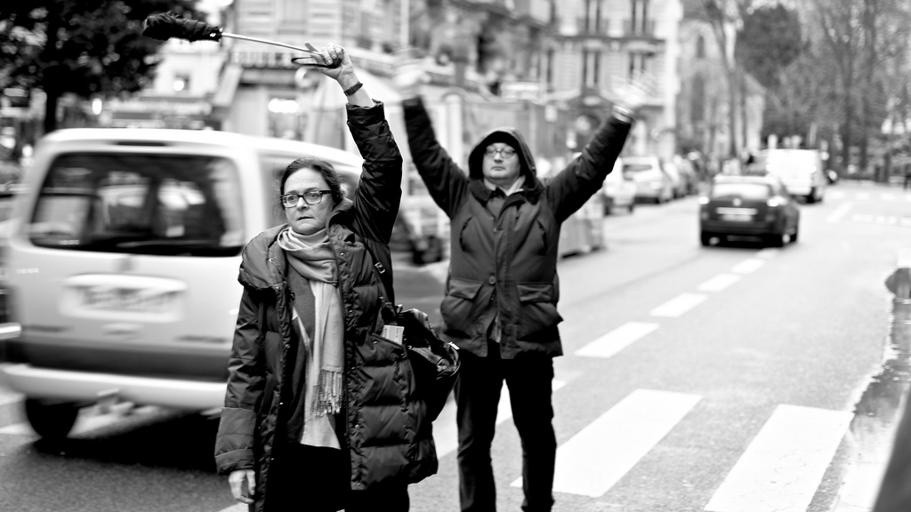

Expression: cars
xmin=536 ymin=161 xmax=604 ymax=257
xmin=606 ymin=153 xmax=698 ymax=214
xmin=699 ymin=175 xmax=798 ymax=247
xmin=756 ymin=148 xmax=824 ymax=204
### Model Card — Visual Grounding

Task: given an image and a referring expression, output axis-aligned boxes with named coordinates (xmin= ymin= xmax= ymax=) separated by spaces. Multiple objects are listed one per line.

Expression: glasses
xmin=280 ymin=187 xmax=333 ymax=208
xmin=484 ymin=145 xmax=518 ymax=159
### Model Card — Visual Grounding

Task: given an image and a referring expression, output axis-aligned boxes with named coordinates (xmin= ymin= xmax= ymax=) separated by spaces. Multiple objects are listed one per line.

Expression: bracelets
xmin=612 ymin=103 xmax=638 ymax=122
xmin=342 ymin=79 xmax=363 ymax=97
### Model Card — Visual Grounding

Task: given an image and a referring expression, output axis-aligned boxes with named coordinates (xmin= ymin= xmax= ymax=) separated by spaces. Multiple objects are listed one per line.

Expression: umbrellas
xmin=137 ymin=10 xmax=341 ymax=70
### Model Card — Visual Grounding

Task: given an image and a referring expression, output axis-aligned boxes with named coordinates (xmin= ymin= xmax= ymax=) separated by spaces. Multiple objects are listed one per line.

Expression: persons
xmin=213 ymin=38 xmax=444 ymax=511
xmin=380 ymin=52 xmax=675 ymax=512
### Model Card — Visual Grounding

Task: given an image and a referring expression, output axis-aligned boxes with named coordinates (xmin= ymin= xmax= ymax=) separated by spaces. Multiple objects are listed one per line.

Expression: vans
xmin=1 ymin=128 xmax=368 ymax=439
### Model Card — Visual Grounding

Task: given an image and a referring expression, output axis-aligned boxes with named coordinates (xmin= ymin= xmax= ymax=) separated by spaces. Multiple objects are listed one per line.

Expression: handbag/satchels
xmin=395 ymin=303 xmax=462 ymax=424
